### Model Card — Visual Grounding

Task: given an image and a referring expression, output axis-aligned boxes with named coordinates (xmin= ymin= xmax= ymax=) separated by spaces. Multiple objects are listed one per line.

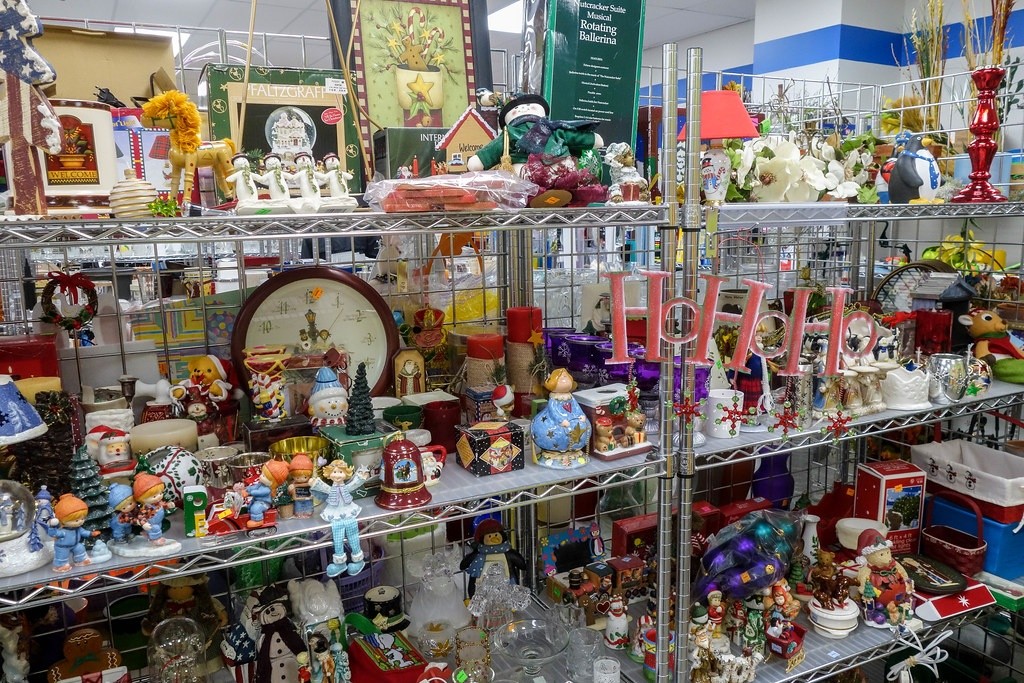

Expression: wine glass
xmin=493 ymin=619 xmax=569 ymax=683
xmin=673 ymin=360 xmax=710 ymax=448
xmin=635 ymin=354 xmax=663 ymax=434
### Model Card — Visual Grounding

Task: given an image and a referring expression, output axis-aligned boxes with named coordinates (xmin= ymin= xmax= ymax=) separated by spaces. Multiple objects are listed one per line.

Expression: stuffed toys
xmin=170 ymin=355 xmax=233 ymax=414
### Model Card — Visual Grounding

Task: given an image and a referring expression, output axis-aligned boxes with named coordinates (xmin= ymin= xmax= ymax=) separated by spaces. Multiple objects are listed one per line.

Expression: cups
xmin=643 ymin=628 xmax=674 ymax=683
xmin=565 ymin=335 xmax=611 ymax=389
xmin=713 ymin=290 xmax=775 ymax=335
xmin=383 ymin=405 xmax=422 ymax=431
xmin=566 ymin=627 xmax=605 ymax=683
xmin=914 ymin=308 xmax=953 ymax=356
xmin=542 ymin=327 xmax=576 ymax=356
xmin=594 ymin=657 xmax=621 ymax=683
xmin=595 ymin=343 xmax=642 ymax=385
xmin=511 ymin=419 xmax=531 ymax=449
xmin=708 ymin=390 xmax=744 ymax=439
xmin=927 ymin=353 xmax=967 ymax=404
xmin=423 ymin=401 xmax=461 ymax=454
xmin=549 ymin=332 xmax=590 ymax=368
xmin=225 ymin=451 xmax=271 ymax=488
xmin=269 ymin=437 xmax=330 ymax=508
xmin=456 ymin=626 xmax=491 ymax=683
xmin=545 ymin=603 xmax=584 ymax=647
xmin=417 ymin=619 xmax=456 ymax=659
xmin=193 ymin=447 xmax=237 ymax=501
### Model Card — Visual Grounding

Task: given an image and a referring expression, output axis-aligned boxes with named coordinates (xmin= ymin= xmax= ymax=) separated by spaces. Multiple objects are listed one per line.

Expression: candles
xmin=466 ymin=332 xmax=507 ymax=388
xmin=506 ymin=305 xmax=546 ymax=416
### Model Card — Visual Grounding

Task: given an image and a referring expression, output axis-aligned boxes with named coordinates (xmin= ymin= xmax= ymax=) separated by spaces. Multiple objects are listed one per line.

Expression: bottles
xmin=803 ymin=515 xmax=820 ymax=567
xmin=129 ymin=280 xmax=140 ymax=301
xmin=702 ymin=138 xmax=731 ymax=207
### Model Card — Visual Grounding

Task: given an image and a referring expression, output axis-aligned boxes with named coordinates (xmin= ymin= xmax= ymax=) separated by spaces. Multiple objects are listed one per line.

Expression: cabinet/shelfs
xmin=0 ymin=0 xmax=673 ymax=683
xmin=536 ymin=0 xmax=1024 ymax=683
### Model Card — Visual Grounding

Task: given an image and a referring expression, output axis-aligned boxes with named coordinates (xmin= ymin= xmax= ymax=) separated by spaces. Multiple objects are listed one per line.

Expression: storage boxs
xmin=316 ymin=417 xmax=401 ymax=499
xmin=911 ymin=441 xmax=1024 ymax=581
xmin=31 ymin=25 xmax=201 ymax=219
xmin=853 ymin=458 xmax=928 ymax=556
xmin=611 ymin=496 xmax=772 ymax=561
xmin=373 ymin=126 xmax=451 ymax=179
xmin=954 ymin=152 xmax=1014 ymax=198
xmin=198 ymin=62 xmax=361 ymax=204
xmin=240 ymin=416 xmax=313 ymax=453
xmin=464 ymin=384 xmax=498 ymax=426
xmin=452 ymin=417 xmax=527 ymax=476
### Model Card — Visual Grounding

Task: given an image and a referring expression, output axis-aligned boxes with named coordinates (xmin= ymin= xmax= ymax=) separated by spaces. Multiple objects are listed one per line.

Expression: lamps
xmin=677 ymin=90 xmax=760 ymax=202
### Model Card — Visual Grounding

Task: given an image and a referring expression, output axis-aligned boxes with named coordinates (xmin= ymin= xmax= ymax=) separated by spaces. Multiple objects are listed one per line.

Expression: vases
xmin=802 ymin=515 xmax=822 ymax=568
xmin=948 ymin=69 xmax=1008 ymax=203
xmin=545 ymin=326 xmax=714 ymax=448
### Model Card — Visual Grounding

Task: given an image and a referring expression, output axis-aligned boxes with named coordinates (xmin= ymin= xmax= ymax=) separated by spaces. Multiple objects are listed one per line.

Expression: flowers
xmin=722 ymin=130 xmax=879 ymax=204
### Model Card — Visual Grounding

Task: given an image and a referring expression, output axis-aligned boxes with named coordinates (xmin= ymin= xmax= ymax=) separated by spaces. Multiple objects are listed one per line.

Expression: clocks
xmin=231 ymin=267 xmax=400 ymax=407
xmin=870 ymin=258 xmax=957 ymax=314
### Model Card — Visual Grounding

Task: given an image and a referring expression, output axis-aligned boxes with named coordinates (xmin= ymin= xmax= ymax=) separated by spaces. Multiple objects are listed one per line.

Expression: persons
xmin=308 ymin=460 xmax=368 ymax=577
xmin=108 ymin=471 xmax=174 ymax=546
xmin=772 ymin=585 xmax=801 ymax=619
xmin=286 ymin=453 xmax=313 ymax=517
xmin=84 ymin=425 xmax=137 ymax=480
xmin=604 ymin=142 xmax=648 ymax=200
xmin=48 ymin=493 xmax=101 ymax=572
xmin=707 ymin=588 xmax=725 ymax=625
xmin=242 ymin=459 xmax=290 ymax=528
xmin=771 ymin=617 xmax=789 ymax=639
xmin=297 ymin=634 xmax=351 ymax=683
xmin=530 ymin=368 xmax=591 ymax=453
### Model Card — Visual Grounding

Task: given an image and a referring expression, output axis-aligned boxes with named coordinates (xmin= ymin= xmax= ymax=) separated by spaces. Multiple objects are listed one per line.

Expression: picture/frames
xmin=330 ymin=0 xmax=493 ymax=194
